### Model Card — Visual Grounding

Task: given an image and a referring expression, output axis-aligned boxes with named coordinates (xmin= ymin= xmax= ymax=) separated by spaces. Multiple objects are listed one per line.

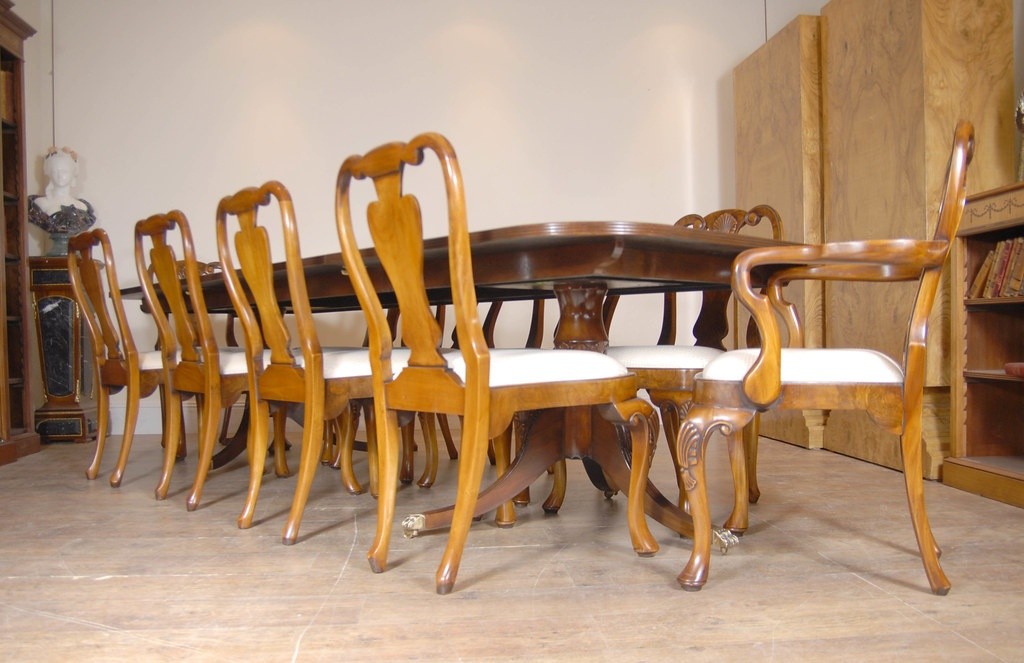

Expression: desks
xmin=109 ymin=221 xmax=813 ymax=557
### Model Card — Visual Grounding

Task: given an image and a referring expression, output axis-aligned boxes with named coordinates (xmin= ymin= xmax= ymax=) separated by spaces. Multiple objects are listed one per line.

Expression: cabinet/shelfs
xmin=1 ymin=1 xmax=41 ymax=467
xmin=732 ymin=1 xmax=1024 ymax=508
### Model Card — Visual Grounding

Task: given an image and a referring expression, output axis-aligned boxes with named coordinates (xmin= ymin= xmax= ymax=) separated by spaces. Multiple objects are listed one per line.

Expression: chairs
xmin=67 ymin=227 xmax=214 ymax=489
xmin=675 ymin=120 xmax=976 ymax=597
xmin=335 ymin=135 xmax=658 ymax=596
xmin=542 ymin=204 xmax=784 ymax=515
xmin=215 ymin=181 xmax=518 ymax=547
xmin=134 ymin=209 xmax=362 ymax=512
xmin=332 ymin=296 xmax=555 ymax=509
xmin=148 ymin=262 xmax=293 ymax=451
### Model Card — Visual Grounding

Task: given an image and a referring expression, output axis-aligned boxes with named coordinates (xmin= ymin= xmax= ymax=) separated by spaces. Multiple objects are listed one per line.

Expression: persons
xmin=34 ymin=149 xmax=87 ymax=216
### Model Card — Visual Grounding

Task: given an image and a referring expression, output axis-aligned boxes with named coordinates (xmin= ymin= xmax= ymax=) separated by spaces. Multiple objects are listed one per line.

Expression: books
xmin=967 ymin=237 xmax=1024 ymax=299
